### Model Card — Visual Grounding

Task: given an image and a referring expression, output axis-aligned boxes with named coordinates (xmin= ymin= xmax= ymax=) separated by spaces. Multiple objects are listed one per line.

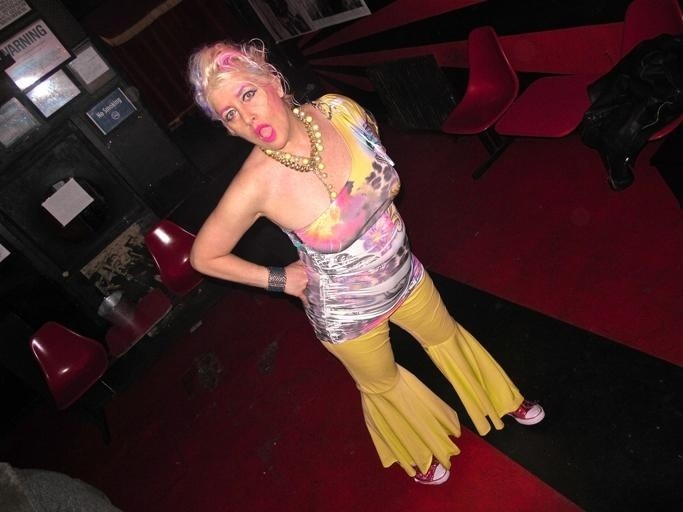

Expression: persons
xmin=187 ymin=37 xmax=547 ymax=487
xmin=0 ymin=462 xmax=122 ymax=512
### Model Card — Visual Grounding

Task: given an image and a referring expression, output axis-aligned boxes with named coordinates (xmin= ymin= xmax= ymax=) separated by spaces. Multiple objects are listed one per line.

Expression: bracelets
xmin=265 ymin=264 xmax=286 ymax=294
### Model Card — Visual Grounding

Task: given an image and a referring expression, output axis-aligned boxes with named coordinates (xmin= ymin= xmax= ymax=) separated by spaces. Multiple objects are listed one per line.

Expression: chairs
xmin=30 ymin=321 xmax=116 ymax=411
xmin=145 ymin=220 xmax=219 ymax=314
xmin=620 ymin=0 xmax=683 ymax=142
xmin=442 ymin=26 xmax=519 ymax=180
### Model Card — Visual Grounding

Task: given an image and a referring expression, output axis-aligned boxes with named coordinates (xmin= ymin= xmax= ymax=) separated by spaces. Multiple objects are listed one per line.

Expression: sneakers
xmin=506 ymin=398 xmax=546 ymax=427
xmin=411 ymin=460 xmax=451 ymax=486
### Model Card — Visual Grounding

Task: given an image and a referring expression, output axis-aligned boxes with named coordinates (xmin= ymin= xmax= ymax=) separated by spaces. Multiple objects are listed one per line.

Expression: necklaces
xmin=257 ymin=107 xmax=338 ymax=201
